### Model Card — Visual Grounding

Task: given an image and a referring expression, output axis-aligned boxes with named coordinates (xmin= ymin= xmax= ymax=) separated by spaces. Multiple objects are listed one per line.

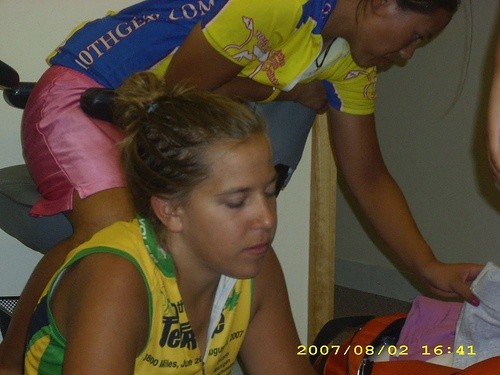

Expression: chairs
xmin=0 ymin=101 xmax=317 ymax=255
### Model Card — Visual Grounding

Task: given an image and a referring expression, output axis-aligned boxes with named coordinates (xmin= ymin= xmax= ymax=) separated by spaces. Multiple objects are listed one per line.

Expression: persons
xmin=22 ymin=71 xmax=316 ymax=375
xmin=0 ymin=1 xmax=486 ymax=375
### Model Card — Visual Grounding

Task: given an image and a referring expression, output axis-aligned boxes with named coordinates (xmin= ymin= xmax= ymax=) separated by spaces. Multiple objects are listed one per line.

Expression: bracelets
xmin=255 ymin=88 xmax=280 ymax=104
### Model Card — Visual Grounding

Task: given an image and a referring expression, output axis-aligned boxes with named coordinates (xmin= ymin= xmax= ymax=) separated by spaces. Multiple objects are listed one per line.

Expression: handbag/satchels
xmin=309 ymin=312 xmax=499 ymax=375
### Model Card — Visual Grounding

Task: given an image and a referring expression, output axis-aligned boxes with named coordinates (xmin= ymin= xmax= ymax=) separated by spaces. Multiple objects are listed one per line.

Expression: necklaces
xmin=313 ymin=38 xmax=336 ymax=69
